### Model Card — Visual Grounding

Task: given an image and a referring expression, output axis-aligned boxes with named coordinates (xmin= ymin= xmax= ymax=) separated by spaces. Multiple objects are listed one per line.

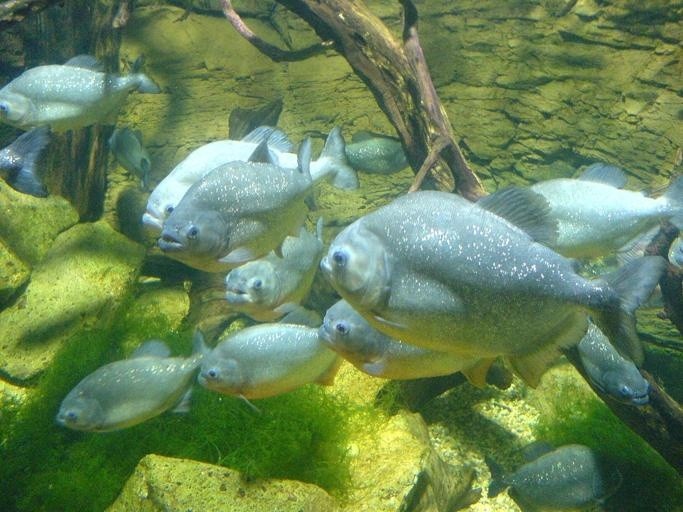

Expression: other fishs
xmin=138 ymin=96 xmax=683 ymax=415
